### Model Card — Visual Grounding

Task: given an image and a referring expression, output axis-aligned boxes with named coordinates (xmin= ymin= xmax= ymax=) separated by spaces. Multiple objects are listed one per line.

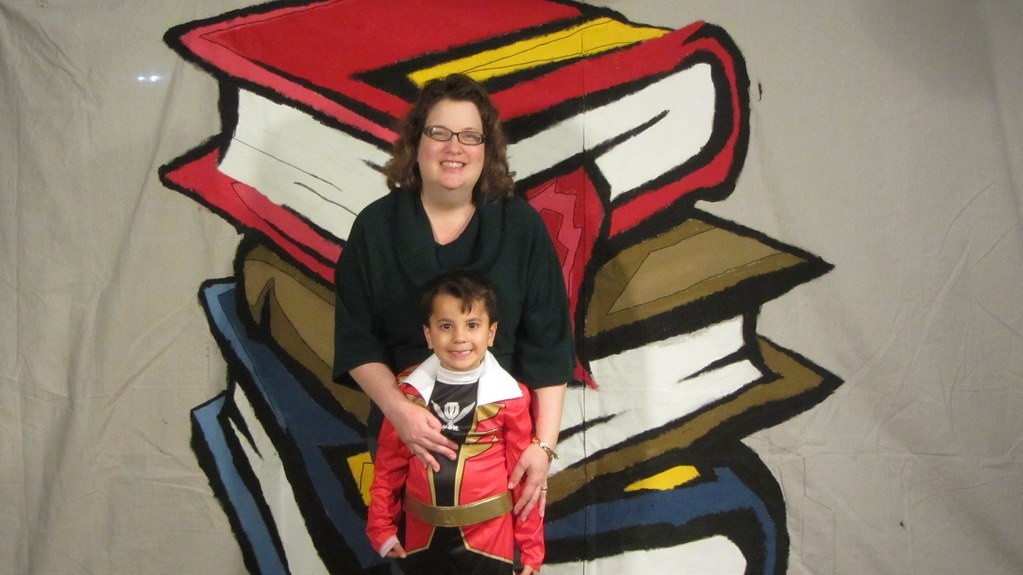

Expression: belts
xmin=402 ymin=491 xmax=514 ymax=527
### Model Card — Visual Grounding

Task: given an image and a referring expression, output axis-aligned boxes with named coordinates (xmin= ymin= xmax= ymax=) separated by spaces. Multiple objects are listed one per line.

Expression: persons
xmin=331 ymin=73 xmax=575 ymax=575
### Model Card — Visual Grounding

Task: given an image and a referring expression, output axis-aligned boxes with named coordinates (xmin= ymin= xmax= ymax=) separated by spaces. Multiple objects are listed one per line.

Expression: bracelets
xmin=532 ymin=435 xmax=559 ymax=462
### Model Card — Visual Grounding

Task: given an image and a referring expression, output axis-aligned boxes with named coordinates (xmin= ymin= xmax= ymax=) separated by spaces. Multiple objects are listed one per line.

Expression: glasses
xmin=422 ymin=126 xmax=486 ymax=145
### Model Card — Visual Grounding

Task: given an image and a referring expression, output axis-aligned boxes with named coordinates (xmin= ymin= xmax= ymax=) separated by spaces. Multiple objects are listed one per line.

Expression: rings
xmin=541 ymin=487 xmax=549 ymax=490
